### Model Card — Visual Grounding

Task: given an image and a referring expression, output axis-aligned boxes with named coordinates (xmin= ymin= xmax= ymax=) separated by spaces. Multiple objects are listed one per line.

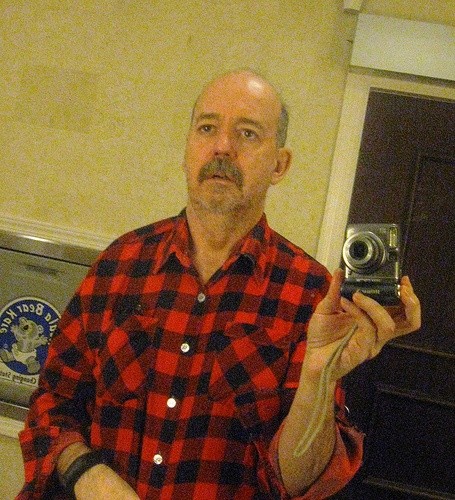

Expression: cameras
xmin=339 ymin=223 xmax=400 ymax=307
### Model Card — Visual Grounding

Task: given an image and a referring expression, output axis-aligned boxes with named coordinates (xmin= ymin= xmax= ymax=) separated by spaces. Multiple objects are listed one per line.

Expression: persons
xmin=14 ymin=68 xmax=421 ymax=500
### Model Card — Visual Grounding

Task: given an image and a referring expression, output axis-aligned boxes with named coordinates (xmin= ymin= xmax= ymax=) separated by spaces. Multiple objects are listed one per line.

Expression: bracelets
xmin=60 ymin=450 xmax=108 ymax=500
